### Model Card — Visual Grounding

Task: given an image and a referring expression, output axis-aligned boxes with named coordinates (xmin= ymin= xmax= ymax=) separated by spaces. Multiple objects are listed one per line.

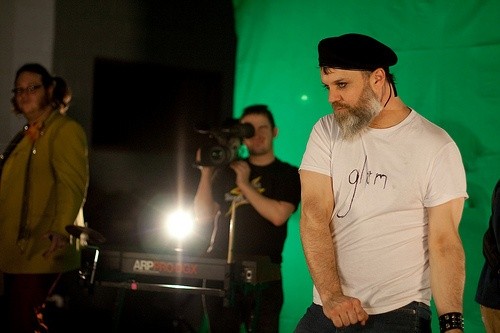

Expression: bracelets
xmin=438 ymin=312 xmax=464 ymax=333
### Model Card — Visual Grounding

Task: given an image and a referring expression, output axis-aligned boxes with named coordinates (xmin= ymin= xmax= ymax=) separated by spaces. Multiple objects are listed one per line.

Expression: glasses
xmin=11 ymin=85 xmax=45 ymax=97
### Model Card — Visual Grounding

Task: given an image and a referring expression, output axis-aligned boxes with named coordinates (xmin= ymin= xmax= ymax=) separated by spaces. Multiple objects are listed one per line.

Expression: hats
xmin=317 ymin=33 xmax=399 ymax=71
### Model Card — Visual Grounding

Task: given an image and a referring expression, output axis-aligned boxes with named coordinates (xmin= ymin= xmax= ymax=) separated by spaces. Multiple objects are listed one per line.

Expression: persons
xmin=192 ymin=104 xmax=301 ymax=333
xmin=0 ymin=74 xmax=73 ymax=175
xmin=292 ymin=33 xmax=470 ymax=333
xmin=474 ymin=178 xmax=500 ymax=333
xmin=0 ymin=62 xmax=90 ymax=333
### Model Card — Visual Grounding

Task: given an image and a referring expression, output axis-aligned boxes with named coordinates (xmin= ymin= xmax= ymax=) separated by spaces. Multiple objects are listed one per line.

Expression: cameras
xmin=196 ymin=119 xmax=253 ymax=167
